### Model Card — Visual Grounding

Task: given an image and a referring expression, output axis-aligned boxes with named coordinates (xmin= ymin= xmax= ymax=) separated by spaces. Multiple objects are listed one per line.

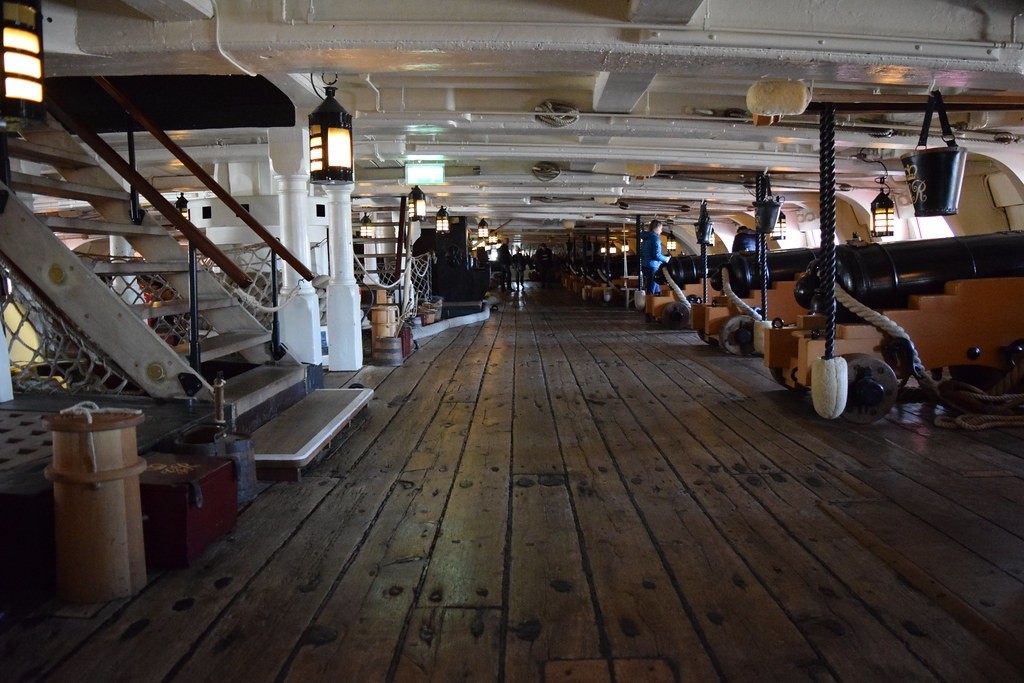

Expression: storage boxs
xmin=139 ymin=452 xmax=238 ymax=570
xmin=399 ymin=326 xmax=411 ymax=358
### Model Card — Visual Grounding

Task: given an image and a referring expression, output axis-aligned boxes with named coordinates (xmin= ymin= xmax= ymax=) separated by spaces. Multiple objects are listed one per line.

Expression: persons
xmin=732 ymin=226 xmax=757 ymax=253
xmin=496 ymin=237 xmax=554 ymax=293
xmin=640 ymin=219 xmax=672 ymax=294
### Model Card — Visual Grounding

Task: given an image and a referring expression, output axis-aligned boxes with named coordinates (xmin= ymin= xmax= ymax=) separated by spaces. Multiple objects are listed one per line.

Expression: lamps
xmin=610 ymin=241 xmax=616 ymax=254
xmin=435 ymin=205 xmax=450 ymax=234
xmin=175 ymin=193 xmax=189 ymax=220
xmin=360 ymin=213 xmax=373 ymax=238
xmin=622 ymin=241 xmax=629 ymax=252
xmin=477 ymin=218 xmax=489 ymax=239
xmin=488 ymin=229 xmax=498 ymax=244
xmin=707 ymin=228 xmax=715 ymax=247
xmin=0 ymin=0 xmax=50 ymax=117
xmin=408 ymin=185 xmax=426 ymax=222
xmin=667 ymin=230 xmax=676 ymax=251
xmin=769 ymin=211 xmax=786 ymax=240
xmin=309 ymin=72 xmax=355 ymax=185
xmin=860 ymin=148 xmax=894 ymax=237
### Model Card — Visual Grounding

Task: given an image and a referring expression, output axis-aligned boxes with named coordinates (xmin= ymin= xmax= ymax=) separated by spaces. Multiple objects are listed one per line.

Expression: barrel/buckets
xmin=374 ymin=337 xmax=403 ymax=367
xmin=174 ymin=423 xmax=257 ymax=502
